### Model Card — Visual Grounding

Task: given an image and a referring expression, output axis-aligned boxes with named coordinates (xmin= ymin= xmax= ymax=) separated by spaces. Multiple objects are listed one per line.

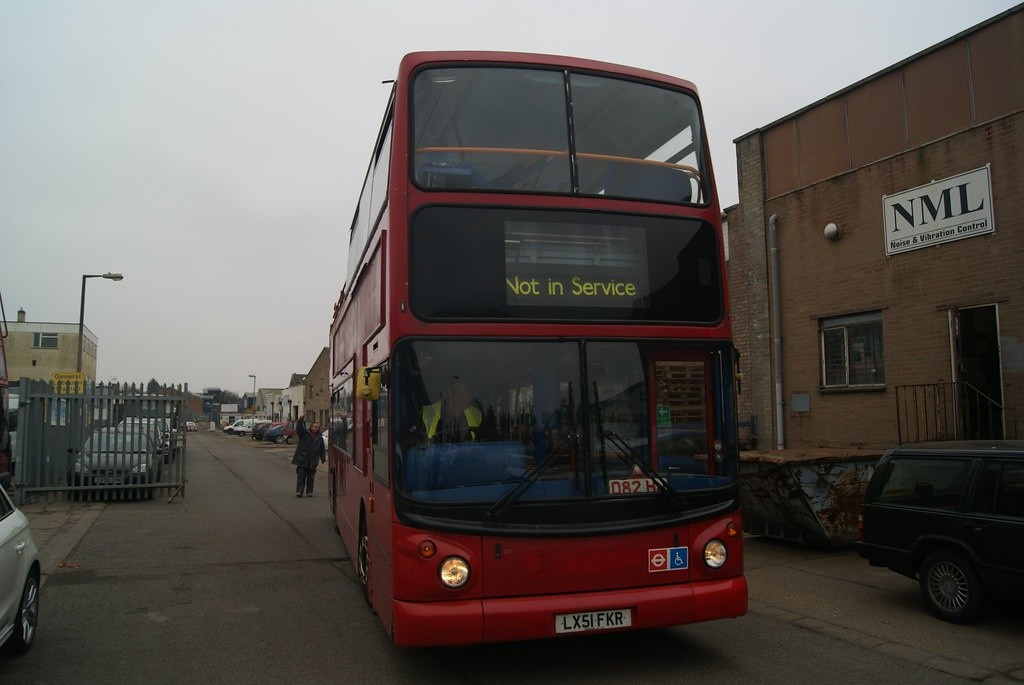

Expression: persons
xmin=292 ymin=416 xmax=326 ymax=497
xmin=410 ymin=369 xmax=490 ymax=449
xmin=332 ymin=412 xmax=348 ymax=493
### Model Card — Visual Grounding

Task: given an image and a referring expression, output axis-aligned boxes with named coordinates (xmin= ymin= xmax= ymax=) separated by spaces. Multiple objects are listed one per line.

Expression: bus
xmin=324 ymin=51 xmax=752 ymax=652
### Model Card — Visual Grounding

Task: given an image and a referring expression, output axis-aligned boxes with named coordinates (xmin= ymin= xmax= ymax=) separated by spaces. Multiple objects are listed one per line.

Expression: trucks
xmin=185 ymin=422 xmax=197 ymax=432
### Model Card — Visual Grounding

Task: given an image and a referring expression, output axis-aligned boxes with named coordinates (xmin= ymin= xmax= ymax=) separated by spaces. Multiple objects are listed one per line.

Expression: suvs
xmin=850 ymin=440 xmax=1024 ymax=623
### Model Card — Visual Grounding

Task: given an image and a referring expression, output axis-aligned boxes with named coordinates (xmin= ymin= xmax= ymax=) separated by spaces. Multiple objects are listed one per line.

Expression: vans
xmin=8 ymin=384 xmax=73 ymax=477
xmin=233 ymin=419 xmax=273 ymax=437
xmin=222 ymin=418 xmax=258 ymax=435
xmin=251 ymin=420 xmax=298 ymax=445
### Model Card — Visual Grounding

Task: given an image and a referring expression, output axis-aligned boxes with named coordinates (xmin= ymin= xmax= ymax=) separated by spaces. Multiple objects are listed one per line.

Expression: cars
xmin=0 ymin=484 xmax=43 ymax=661
xmin=119 ymin=415 xmax=175 ymax=464
xmin=322 ymin=429 xmax=328 ymax=452
xmin=67 ymin=425 xmax=163 ymax=501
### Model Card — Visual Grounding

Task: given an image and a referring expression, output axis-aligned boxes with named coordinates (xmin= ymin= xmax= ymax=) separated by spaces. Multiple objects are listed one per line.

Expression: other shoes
xmin=307 ymin=491 xmax=313 ymax=497
xmin=296 ymin=491 xmax=306 ymax=495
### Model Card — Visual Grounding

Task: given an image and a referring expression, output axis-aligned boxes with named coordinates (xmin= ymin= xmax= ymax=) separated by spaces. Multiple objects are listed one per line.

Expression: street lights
xmin=77 ymin=272 xmax=125 ymax=373
xmin=248 ymin=374 xmax=256 ymax=439
